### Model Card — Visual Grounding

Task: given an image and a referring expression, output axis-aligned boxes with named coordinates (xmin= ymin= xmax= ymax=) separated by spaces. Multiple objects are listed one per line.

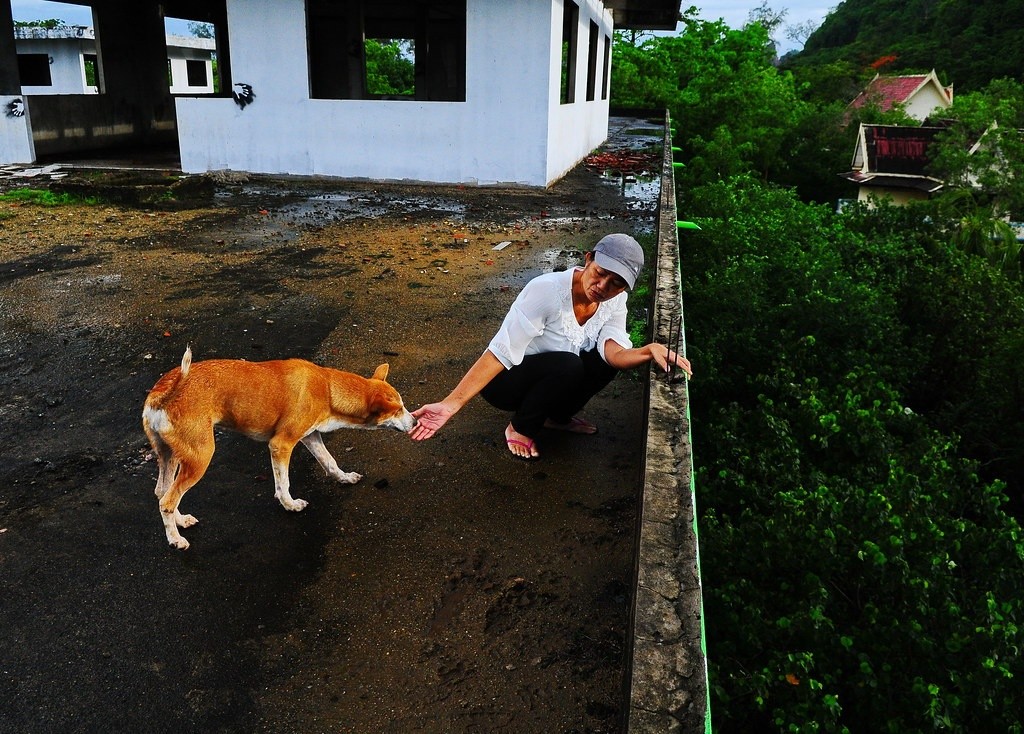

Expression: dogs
xmin=142 ymin=343 xmax=418 ymax=550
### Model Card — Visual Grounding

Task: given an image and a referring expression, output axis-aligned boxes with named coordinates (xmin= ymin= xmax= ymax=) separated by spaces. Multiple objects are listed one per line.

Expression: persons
xmin=408 ymin=233 xmax=692 ymax=461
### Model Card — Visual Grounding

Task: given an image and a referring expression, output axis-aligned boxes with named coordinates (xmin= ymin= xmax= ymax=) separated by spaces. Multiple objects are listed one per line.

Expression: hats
xmin=593 ymin=233 xmax=645 ymax=290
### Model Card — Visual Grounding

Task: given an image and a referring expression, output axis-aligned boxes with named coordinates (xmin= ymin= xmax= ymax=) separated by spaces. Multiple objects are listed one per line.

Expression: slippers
xmin=504 ymin=434 xmax=541 ymax=461
xmin=546 ymin=417 xmax=600 ymax=436
